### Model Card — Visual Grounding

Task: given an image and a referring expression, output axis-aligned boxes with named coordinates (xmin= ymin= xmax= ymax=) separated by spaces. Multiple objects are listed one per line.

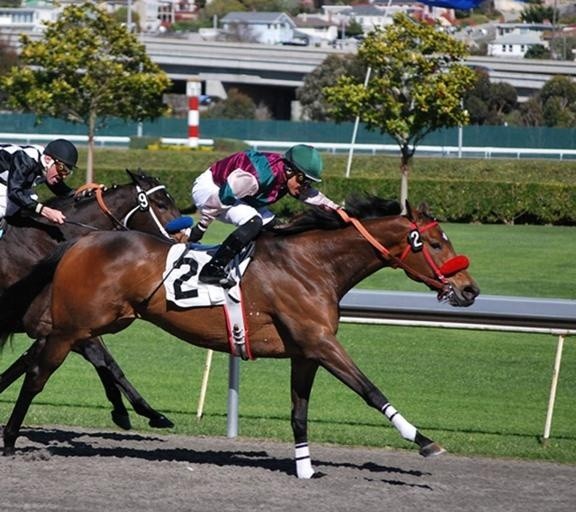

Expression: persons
xmin=187 ymin=144 xmax=346 ymax=288
xmin=0 ymin=139 xmax=78 ymax=240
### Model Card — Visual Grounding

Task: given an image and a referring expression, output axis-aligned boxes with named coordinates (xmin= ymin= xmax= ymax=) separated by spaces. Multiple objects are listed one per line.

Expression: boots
xmin=199 ymin=234 xmax=245 ymax=288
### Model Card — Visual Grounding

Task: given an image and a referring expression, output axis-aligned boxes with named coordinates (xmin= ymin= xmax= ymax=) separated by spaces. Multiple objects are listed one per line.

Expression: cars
xmin=198 ymin=95 xmax=223 ymax=113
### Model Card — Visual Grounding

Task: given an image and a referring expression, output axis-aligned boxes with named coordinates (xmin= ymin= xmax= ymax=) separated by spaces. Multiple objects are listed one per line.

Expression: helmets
xmin=284 ymin=144 xmax=322 ymax=183
xmin=43 ymin=140 xmax=78 ymax=170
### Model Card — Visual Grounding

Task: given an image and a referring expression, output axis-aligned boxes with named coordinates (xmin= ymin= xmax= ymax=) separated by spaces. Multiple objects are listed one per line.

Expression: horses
xmin=0 ymin=188 xmax=481 ymax=480
xmin=0 ymin=166 xmax=185 ymax=432
